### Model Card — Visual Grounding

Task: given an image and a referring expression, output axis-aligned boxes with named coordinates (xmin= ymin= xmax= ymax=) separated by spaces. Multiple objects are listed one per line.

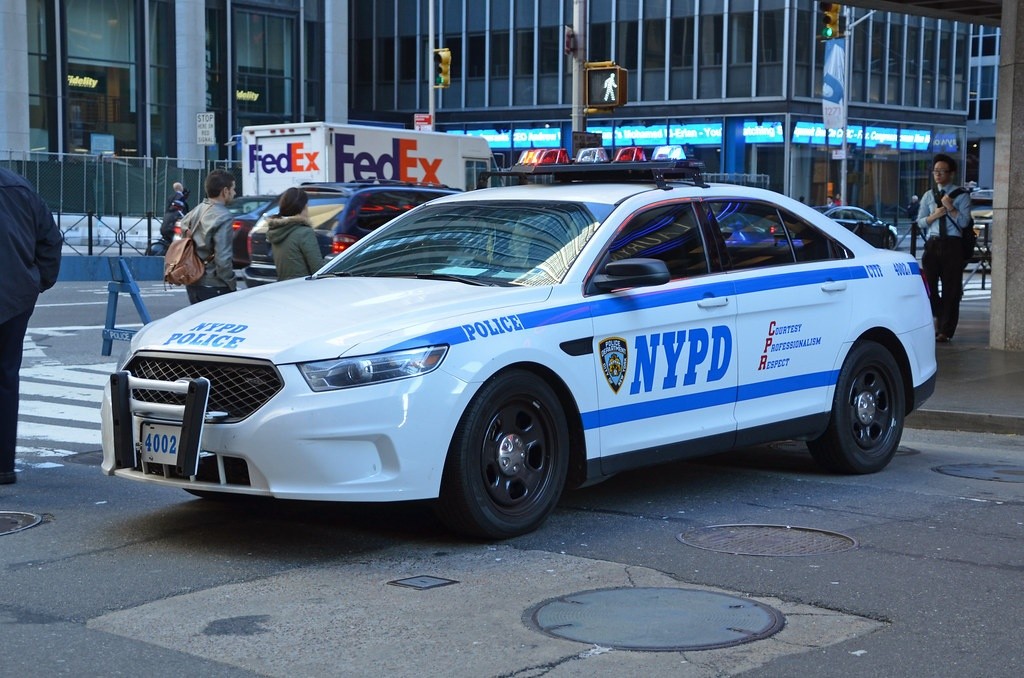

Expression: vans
xmin=242 ymin=118 xmax=504 ymax=231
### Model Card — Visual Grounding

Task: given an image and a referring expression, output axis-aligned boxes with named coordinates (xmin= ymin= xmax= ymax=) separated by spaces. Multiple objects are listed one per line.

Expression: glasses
xmin=931 ymin=169 xmax=952 ymax=174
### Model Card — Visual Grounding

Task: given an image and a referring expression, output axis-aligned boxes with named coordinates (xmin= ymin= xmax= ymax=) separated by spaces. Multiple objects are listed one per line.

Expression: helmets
xmin=172 ymin=200 xmax=185 ymax=207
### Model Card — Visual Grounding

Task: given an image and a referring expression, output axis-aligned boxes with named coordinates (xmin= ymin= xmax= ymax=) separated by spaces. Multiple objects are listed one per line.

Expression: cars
xmin=221 ymin=194 xmax=280 ymax=269
xmin=766 ymin=195 xmax=899 ymax=253
xmin=101 ymin=160 xmax=939 ymax=546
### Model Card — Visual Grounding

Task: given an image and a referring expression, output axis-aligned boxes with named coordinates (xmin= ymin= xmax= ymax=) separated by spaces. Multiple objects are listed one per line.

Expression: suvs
xmin=242 ymin=180 xmax=467 ymax=294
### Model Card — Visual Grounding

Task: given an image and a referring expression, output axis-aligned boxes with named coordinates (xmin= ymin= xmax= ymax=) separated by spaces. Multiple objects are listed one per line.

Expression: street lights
xmin=819 ymin=1 xmax=840 ymax=40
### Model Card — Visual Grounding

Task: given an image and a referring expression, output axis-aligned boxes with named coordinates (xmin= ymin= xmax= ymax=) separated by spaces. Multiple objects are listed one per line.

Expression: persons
xmin=797 ymin=193 xmax=844 ymax=209
xmin=264 ymin=187 xmax=324 ymax=283
xmin=919 ymin=154 xmax=972 ymax=342
xmin=0 ymin=164 xmax=64 ymax=486
xmin=176 ymin=169 xmax=237 ymax=306
xmin=160 ymin=182 xmax=189 ymax=244
xmin=908 ymin=195 xmax=920 ymax=221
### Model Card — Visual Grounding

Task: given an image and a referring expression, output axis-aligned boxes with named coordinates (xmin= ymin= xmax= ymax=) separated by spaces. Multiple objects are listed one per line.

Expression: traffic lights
xmin=585 ymin=66 xmax=630 ymax=109
xmin=433 ymin=47 xmax=452 ymax=89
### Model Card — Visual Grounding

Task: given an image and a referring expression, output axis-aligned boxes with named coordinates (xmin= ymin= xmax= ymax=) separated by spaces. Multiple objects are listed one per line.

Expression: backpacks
xmin=164 ymin=205 xmax=216 ymax=285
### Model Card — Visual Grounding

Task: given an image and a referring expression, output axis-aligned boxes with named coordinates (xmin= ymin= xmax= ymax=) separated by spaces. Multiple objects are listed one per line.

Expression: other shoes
xmin=2 ymin=471 xmax=17 ymax=483
xmin=936 ymin=332 xmax=951 ymax=342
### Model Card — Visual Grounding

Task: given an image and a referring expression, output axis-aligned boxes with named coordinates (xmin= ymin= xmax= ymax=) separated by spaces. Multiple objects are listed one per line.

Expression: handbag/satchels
xmin=946 ymin=187 xmax=976 ymax=245
